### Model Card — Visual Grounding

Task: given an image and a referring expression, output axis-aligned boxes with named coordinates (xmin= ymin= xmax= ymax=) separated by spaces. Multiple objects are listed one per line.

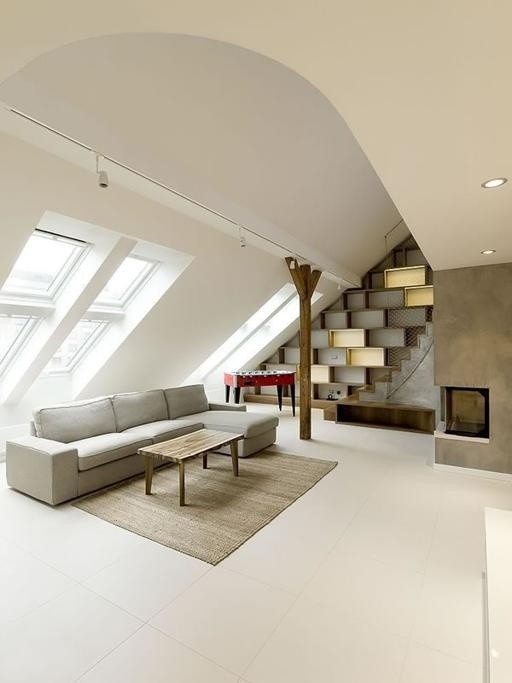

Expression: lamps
xmin=235 ymin=227 xmax=247 ymax=248
xmin=90 ymin=152 xmax=109 ymax=189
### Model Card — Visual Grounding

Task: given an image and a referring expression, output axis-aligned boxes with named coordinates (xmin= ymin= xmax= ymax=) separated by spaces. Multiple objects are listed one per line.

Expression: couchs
xmin=3 ymin=383 xmax=279 ymax=507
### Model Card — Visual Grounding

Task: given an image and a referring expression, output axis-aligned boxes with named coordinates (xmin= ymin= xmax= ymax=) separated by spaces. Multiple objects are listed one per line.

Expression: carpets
xmin=70 ymin=449 xmax=339 ymax=568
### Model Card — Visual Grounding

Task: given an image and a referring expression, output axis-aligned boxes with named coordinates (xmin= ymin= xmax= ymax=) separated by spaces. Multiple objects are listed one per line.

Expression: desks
xmin=223 ymin=370 xmax=298 ymax=420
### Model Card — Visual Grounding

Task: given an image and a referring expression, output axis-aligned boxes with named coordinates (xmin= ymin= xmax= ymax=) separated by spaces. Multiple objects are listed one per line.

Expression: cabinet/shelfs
xmin=246 ymin=232 xmax=435 ymax=409
